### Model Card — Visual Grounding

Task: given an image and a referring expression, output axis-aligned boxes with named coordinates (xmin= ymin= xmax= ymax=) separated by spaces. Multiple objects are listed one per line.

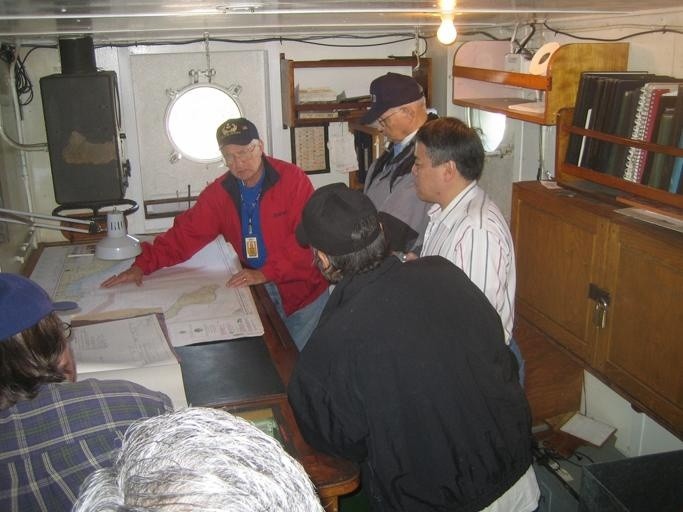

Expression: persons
xmin=360 ymin=70 xmax=441 ymax=262
xmin=0 ymin=271 xmax=175 ymax=512
xmin=71 ymin=404 xmax=326 ymax=512
xmin=287 ymin=182 xmax=542 ymax=512
xmin=411 ymin=116 xmax=527 ymax=389
xmin=100 ymin=118 xmax=331 ymax=353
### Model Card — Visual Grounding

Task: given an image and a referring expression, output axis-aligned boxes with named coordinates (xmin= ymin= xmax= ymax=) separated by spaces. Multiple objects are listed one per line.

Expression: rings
xmin=243 ymin=277 xmax=247 ymax=281
xmin=113 ymin=273 xmax=120 ymax=277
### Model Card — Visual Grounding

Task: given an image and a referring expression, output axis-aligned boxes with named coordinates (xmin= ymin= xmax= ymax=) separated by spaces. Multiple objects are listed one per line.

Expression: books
xmin=564 ymin=70 xmax=683 ymax=195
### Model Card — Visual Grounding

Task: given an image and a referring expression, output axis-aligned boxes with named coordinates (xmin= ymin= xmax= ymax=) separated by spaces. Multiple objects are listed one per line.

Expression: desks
xmin=21 ymin=229 xmax=361 ymax=512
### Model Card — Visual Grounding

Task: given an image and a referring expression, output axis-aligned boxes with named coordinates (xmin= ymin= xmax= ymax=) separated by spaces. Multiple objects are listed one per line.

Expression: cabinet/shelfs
xmin=451 ymin=37 xmax=682 ymax=223
xmin=509 ymin=177 xmax=682 ymax=443
xmin=279 ymin=54 xmax=433 ymax=131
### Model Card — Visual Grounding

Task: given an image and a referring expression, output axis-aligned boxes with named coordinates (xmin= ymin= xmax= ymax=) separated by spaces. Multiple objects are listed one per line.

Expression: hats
xmin=217 ymin=118 xmax=260 ymax=153
xmin=360 ymin=74 xmax=424 ymax=127
xmin=295 ymin=182 xmax=382 ymax=255
xmin=0 ymin=271 xmax=77 ymax=341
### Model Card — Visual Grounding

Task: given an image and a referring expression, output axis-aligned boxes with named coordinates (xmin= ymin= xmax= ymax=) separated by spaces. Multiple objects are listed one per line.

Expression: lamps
xmin=432 ymin=10 xmax=463 ymax=45
xmin=0 ymin=200 xmax=145 ymax=262
